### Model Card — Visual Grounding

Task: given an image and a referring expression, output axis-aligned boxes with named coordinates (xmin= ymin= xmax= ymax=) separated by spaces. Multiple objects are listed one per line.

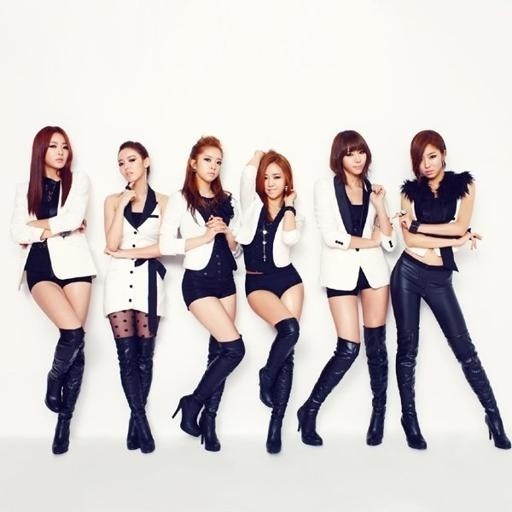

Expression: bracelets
xmin=283 ymin=206 xmax=295 ymax=216
xmin=408 ymin=220 xmax=420 ymax=233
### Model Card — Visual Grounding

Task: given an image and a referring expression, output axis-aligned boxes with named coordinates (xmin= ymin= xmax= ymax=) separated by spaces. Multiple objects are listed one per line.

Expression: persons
xmin=103 ymin=141 xmax=170 ymax=453
xmin=239 ymin=150 xmax=304 ymax=453
xmin=297 ymin=130 xmax=397 ymax=445
xmin=390 ymin=130 xmax=512 ymax=450
xmin=159 ymin=137 xmax=245 ymax=451
xmin=10 ymin=126 xmax=97 ymax=453
